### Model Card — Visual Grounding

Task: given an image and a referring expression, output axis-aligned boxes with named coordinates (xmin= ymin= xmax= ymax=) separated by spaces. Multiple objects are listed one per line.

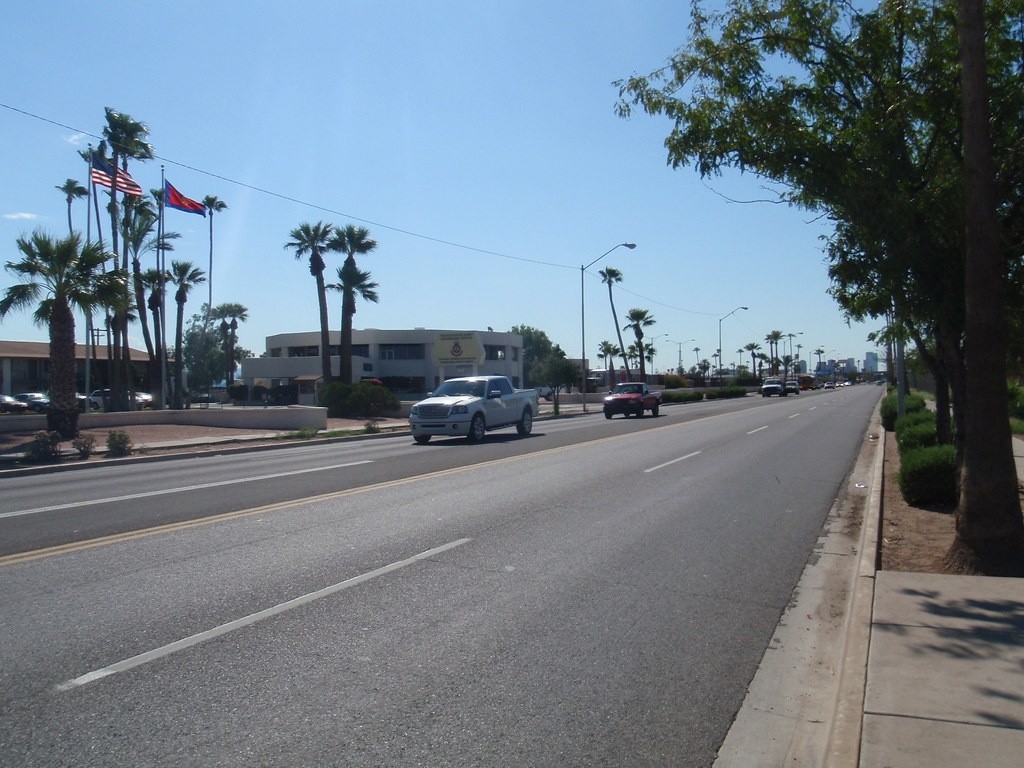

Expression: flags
xmin=164 ymin=179 xmax=206 ymax=218
xmin=91 ymin=154 xmax=141 ymax=196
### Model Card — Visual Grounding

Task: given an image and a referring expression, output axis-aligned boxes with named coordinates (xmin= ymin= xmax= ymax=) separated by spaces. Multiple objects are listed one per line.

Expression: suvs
xmin=760 ymin=378 xmax=788 ymax=398
xmin=89 ymin=386 xmax=144 ymax=412
xmin=44 ymin=389 xmax=91 ymax=409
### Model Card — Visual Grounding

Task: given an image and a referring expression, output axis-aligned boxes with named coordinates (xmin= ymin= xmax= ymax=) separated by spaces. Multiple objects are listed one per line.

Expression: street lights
xmin=783 ymin=332 xmax=804 ymax=358
xmin=718 ymin=306 xmax=749 ymax=390
xmin=824 ymin=349 xmax=836 ymax=362
xmin=665 ymin=338 xmax=696 ymax=386
xmin=809 ymin=344 xmax=825 ymax=375
xmin=651 ymin=332 xmax=669 ymax=375
xmin=580 ymin=243 xmax=637 ymax=411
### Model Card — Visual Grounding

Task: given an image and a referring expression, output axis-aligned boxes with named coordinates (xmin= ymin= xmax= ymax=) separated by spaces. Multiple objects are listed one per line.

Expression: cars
xmin=786 ymin=381 xmax=800 ymax=394
xmin=0 ymin=393 xmax=29 ymax=415
xmin=15 ymin=392 xmax=51 ymax=413
xmin=135 ymin=391 xmax=154 ymax=408
xmin=798 ymin=373 xmax=852 ymax=392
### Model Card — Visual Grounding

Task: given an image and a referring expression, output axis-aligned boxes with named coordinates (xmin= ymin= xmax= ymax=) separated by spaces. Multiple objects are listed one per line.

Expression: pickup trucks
xmin=602 ymin=381 xmax=664 ymax=420
xmin=532 ymin=384 xmax=561 ymax=401
xmin=408 ymin=376 xmax=540 ymax=445
